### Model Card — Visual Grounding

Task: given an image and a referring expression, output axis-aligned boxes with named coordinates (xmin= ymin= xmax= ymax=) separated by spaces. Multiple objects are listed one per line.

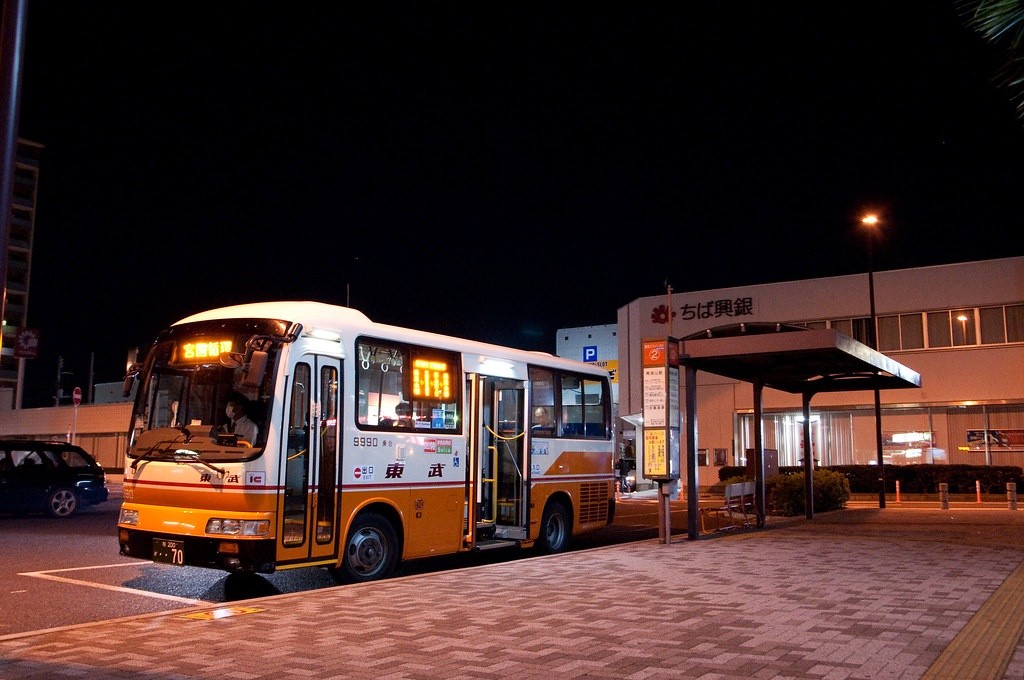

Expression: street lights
xmin=862 ymin=215 xmax=890 ymax=508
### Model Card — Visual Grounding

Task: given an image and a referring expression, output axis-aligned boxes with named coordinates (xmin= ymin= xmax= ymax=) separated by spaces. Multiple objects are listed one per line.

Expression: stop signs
xmin=73 ymin=387 xmax=83 ymax=408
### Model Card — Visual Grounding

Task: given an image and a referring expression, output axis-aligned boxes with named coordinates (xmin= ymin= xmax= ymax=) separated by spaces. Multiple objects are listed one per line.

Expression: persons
xmin=218 ymin=389 xmax=258 ymax=446
xmin=615 ymin=458 xmax=631 ymax=495
xmin=396 ymin=403 xmax=413 ymax=428
xmin=532 ymin=407 xmax=554 ymax=427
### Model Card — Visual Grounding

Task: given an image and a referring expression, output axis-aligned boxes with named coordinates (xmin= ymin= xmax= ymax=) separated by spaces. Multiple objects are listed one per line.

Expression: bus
xmin=116 ymin=300 xmax=619 ymax=590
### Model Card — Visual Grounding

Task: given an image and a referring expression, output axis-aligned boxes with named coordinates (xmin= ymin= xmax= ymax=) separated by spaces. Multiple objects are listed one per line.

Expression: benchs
xmin=699 ymin=482 xmax=763 ymax=535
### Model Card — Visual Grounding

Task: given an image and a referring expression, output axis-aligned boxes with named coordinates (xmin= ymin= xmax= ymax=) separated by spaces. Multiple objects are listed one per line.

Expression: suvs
xmin=1 ymin=438 xmax=109 ymax=519
xmin=614 ymin=457 xmax=637 ymax=493
